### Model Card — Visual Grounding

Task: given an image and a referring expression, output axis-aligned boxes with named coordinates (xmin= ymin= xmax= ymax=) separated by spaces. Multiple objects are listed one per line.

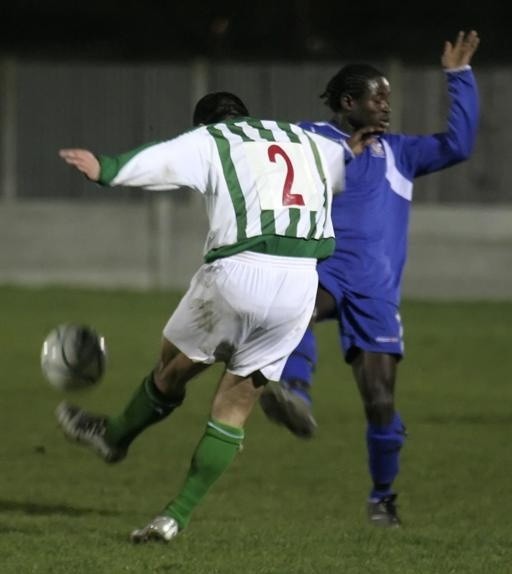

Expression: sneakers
xmin=56 ymin=398 xmax=126 ymax=464
xmin=131 ymin=515 xmax=179 ymax=545
xmin=260 ymin=381 xmax=318 ymax=440
xmin=368 ymin=493 xmax=402 ymax=528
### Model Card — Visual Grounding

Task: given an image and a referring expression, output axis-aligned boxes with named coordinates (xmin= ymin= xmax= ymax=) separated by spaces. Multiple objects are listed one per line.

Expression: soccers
xmin=41 ymin=325 xmax=105 ymax=389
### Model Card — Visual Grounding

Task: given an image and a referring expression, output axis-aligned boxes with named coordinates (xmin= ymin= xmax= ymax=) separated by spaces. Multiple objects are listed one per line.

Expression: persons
xmin=54 ymin=92 xmax=346 ymax=544
xmin=257 ymin=28 xmax=481 ymax=529
